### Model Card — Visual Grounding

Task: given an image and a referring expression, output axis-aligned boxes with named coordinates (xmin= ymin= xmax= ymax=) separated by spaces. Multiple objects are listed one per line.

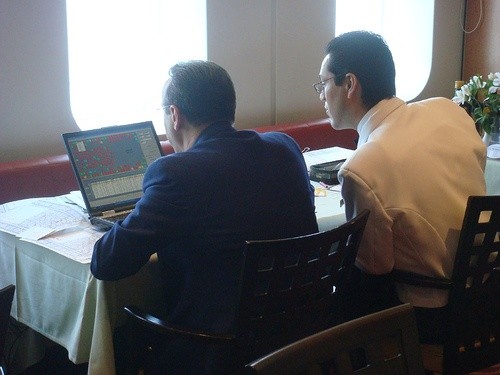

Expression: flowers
xmin=450 ymin=72 xmax=500 ymax=135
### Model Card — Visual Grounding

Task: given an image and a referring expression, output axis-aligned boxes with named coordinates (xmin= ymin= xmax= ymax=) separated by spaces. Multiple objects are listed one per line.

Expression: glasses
xmin=313 ymin=73 xmax=360 ymax=95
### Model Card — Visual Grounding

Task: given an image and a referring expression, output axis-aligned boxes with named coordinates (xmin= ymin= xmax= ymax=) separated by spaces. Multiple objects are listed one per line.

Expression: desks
xmin=0 ymin=146 xmax=499 ymax=375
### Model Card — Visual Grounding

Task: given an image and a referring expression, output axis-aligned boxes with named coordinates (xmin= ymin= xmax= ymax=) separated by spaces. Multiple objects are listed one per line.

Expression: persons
xmin=314 ymin=30 xmax=500 ymax=343
xmin=90 ymin=60 xmax=328 ymax=375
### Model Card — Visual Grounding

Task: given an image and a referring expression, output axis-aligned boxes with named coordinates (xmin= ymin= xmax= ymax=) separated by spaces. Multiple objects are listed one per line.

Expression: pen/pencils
xmin=320 ymin=182 xmax=328 ymax=188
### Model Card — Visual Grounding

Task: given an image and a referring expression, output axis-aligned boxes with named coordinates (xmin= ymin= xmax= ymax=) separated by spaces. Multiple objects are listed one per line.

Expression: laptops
xmin=61 ymin=121 xmax=166 ymax=230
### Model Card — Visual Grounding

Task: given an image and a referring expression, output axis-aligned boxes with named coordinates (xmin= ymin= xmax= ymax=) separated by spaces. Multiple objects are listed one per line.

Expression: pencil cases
xmin=309 ymin=159 xmax=346 ymax=185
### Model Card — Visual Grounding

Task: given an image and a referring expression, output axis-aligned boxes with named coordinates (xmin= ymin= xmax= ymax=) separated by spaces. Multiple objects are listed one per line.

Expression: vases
xmin=481 ymin=117 xmax=500 ymax=148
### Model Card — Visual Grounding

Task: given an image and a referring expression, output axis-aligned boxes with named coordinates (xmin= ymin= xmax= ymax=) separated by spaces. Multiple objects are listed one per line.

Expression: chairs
xmin=119 ymin=208 xmax=371 ymax=375
xmin=244 ymin=302 xmax=425 ymax=375
xmin=356 ymin=195 xmax=500 ymax=375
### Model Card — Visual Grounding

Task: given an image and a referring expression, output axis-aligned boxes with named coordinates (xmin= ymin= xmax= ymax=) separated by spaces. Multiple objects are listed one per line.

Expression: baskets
xmin=310 ymin=158 xmax=347 ymax=184
